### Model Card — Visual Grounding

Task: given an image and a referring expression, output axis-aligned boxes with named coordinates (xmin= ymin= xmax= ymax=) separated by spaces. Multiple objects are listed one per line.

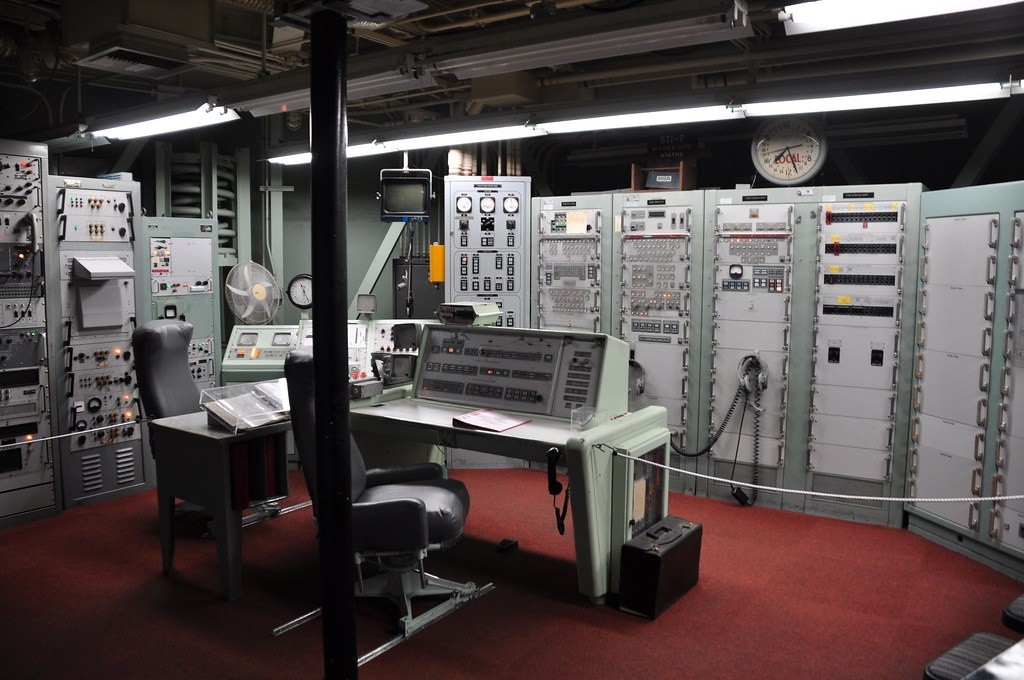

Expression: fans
xmin=224 ymin=260 xmax=283 ymax=325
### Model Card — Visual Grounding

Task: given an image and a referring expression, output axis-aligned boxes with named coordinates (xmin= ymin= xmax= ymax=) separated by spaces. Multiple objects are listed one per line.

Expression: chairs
xmin=270 ymin=350 xmax=498 ymax=668
xmin=131 ymin=318 xmax=280 ymax=518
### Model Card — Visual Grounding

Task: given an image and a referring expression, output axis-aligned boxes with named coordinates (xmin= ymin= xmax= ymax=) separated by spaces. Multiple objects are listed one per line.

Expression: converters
xmin=728 ymin=486 xmax=750 ymax=507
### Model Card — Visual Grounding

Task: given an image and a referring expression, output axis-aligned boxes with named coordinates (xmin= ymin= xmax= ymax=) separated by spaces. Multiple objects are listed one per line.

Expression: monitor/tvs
xmin=377 ymin=175 xmax=431 ymax=224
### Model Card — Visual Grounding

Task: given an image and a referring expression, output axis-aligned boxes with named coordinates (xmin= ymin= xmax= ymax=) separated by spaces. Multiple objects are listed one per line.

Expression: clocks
xmin=750 ymin=113 xmax=828 ymax=186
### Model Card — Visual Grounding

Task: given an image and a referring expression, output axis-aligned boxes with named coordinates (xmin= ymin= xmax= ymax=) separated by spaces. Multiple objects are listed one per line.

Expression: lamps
xmin=74 ymin=95 xmax=244 ymax=146
xmin=257 ymin=77 xmax=1024 ymax=170
xmin=238 ymin=62 xmax=440 ymax=120
xmin=436 ymin=1 xmax=758 ymax=81
xmin=777 ymin=0 xmax=1024 ymax=37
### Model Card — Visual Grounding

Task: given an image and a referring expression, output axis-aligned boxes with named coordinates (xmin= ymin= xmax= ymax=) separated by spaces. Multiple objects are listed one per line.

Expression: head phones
xmin=627 ymin=359 xmax=646 ymax=395
xmin=735 ymin=355 xmax=768 ymax=394
